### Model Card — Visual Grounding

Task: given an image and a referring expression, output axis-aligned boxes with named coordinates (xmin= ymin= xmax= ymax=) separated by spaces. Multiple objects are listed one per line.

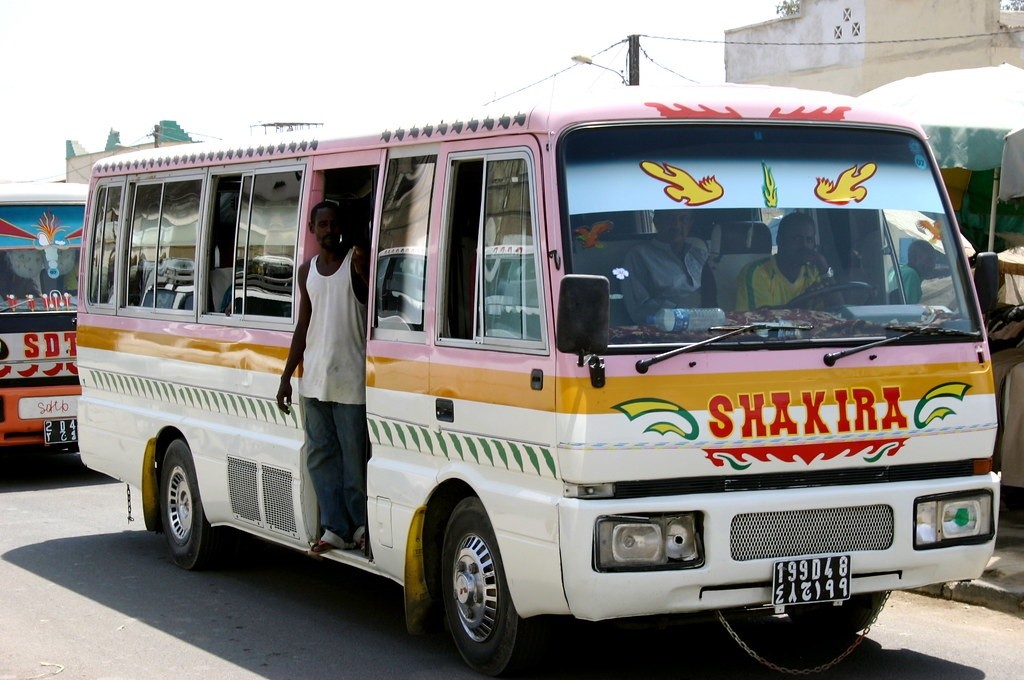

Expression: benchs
xmin=505 ymin=229 xmax=708 ymax=325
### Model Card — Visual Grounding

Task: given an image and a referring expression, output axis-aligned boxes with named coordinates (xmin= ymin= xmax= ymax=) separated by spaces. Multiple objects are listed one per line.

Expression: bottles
xmin=646 ymin=307 xmax=725 ymax=333
xmin=750 ymin=320 xmax=801 ymax=337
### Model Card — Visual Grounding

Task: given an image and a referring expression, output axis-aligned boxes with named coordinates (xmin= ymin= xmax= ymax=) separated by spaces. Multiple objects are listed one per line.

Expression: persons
xmin=887 ymin=239 xmax=936 ymax=305
xmin=276 ymin=202 xmax=369 ymax=556
xmin=620 ymin=210 xmax=709 ymax=325
xmin=737 ymin=212 xmax=845 ymax=311
xmin=221 ymin=245 xmax=280 ymax=316
xmin=0 ymin=251 xmax=79 ymax=305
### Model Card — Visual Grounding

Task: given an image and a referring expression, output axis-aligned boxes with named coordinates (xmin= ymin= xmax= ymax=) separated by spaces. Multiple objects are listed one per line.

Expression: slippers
xmin=310 ymin=539 xmax=335 ymax=553
xmin=360 ymin=533 xmax=367 ymax=555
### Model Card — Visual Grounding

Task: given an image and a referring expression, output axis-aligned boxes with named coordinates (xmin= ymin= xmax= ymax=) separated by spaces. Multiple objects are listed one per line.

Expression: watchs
xmin=821 ymin=267 xmax=834 ymax=280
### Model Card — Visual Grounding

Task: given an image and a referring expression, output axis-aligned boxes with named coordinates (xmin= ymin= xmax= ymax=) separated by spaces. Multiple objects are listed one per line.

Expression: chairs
xmin=141 ymin=263 xmax=246 ymax=315
xmin=699 ymin=220 xmax=772 ymax=309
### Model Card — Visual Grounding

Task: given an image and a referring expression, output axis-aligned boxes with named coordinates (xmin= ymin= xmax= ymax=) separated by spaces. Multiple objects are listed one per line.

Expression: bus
xmin=0 ymin=181 xmax=119 ymax=488
xmin=72 ymin=84 xmax=1003 ymax=680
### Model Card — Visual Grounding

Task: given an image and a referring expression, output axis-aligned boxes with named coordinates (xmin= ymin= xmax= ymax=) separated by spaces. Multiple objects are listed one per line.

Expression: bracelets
xmin=281 ymin=374 xmax=291 ymax=380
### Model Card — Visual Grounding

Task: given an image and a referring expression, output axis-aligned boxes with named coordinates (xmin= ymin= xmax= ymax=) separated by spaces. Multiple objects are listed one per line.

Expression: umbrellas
xmin=856 ymin=62 xmax=1024 ymax=252
